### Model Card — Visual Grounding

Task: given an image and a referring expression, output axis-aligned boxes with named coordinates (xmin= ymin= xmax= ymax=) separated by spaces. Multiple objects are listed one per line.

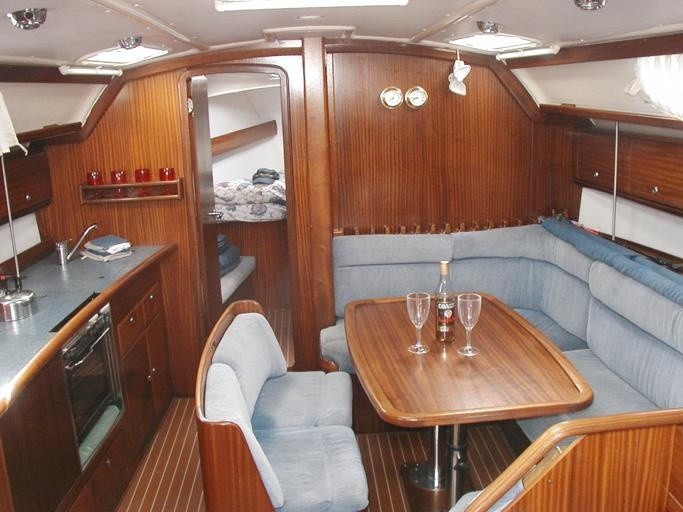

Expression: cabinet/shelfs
xmin=0 ymin=349 xmax=83 ymax=511
xmin=115 ymin=280 xmax=173 ymax=464
xmin=78 ymin=176 xmax=183 ymax=205
xmin=572 ymin=129 xmax=683 ymax=217
xmin=69 ymin=480 xmax=96 ymax=512
xmin=89 ymin=428 xmax=136 ymax=512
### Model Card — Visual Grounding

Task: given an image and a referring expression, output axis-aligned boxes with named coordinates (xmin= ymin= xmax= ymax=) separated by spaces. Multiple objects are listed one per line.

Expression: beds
xmin=214 ymin=170 xmax=286 ymax=223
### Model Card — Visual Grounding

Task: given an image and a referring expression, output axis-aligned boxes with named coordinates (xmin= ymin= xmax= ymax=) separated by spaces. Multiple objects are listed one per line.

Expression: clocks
xmin=379 ymin=86 xmax=404 ymax=110
xmin=405 ymin=86 xmax=429 ymax=110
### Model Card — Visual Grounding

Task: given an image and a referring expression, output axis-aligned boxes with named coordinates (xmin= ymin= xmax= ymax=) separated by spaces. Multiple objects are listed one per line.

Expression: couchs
xmin=195 ymin=299 xmax=371 ymax=511
xmin=319 ymin=215 xmax=683 ymax=511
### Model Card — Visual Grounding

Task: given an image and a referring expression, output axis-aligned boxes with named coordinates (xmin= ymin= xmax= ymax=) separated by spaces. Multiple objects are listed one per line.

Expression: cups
xmin=88 ymin=170 xmax=105 ymax=199
xmin=112 ymin=171 xmax=127 ymax=198
xmin=159 ymin=167 xmax=178 ymax=195
xmin=135 ymin=169 xmax=151 ymax=196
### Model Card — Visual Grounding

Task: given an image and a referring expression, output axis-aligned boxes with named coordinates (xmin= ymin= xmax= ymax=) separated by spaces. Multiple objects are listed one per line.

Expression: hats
xmin=253 ymin=168 xmax=279 ymax=185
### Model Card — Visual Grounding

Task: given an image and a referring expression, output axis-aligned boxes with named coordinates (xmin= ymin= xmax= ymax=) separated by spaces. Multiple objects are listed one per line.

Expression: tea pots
xmin=0 ymin=276 xmax=38 ymax=322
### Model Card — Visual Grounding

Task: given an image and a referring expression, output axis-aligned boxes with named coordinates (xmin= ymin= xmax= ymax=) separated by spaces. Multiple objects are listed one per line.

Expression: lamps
xmin=477 ymin=21 xmax=502 ymax=35
xmin=117 ymin=35 xmax=142 ymax=50
xmin=6 ymin=8 xmax=47 ymax=30
xmin=448 ymin=48 xmax=471 ymax=96
xmin=574 ymin=0 xmax=606 ymax=10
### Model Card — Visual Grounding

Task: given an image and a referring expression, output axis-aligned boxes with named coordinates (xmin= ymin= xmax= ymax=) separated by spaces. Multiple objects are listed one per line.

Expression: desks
xmin=344 ymin=291 xmax=594 ymax=511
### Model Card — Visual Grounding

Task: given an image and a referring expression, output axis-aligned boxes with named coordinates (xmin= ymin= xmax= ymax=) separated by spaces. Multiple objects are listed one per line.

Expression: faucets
xmin=67 ymin=224 xmax=99 ymax=260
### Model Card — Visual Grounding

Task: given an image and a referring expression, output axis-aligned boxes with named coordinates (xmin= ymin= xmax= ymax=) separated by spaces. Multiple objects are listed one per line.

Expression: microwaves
xmin=62 ymin=303 xmax=125 ymax=474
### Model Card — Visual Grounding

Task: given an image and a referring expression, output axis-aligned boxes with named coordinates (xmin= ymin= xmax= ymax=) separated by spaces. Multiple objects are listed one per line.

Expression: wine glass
xmin=456 ymin=294 xmax=482 ymax=356
xmin=407 ymin=292 xmax=430 ymax=354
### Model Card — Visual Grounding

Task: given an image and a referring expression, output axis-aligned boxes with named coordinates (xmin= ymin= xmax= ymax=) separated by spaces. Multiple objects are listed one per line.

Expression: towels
xmin=80 ymin=234 xmax=132 ymax=262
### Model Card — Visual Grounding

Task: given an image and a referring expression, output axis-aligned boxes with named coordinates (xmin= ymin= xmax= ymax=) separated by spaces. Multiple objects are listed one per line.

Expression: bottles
xmin=434 ymin=261 xmax=456 ymax=345
